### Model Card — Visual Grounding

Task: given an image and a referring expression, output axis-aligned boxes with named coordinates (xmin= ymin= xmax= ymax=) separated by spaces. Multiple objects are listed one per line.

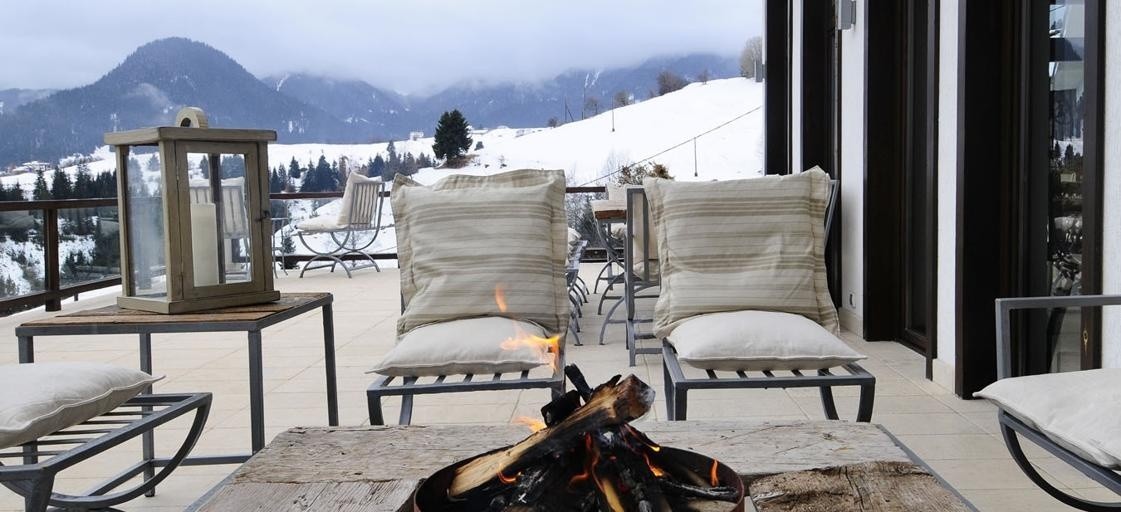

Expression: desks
xmin=187 ymin=425 xmax=975 ymax=511
xmin=14 ymin=292 xmax=338 ymax=498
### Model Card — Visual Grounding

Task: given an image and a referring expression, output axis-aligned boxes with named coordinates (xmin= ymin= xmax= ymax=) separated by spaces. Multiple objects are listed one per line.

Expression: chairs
xmin=657 ymin=178 xmax=875 ymax=424
xmin=290 ymin=179 xmax=382 ymax=279
xmin=567 ymin=191 xmax=657 ymax=349
xmin=364 ymin=283 xmax=568 ymax=426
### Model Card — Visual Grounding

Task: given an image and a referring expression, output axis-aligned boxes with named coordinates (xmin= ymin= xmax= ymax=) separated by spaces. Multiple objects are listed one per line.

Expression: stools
xmin=1 ymin=361 xmax=213 ymax=510
xmin=971 ymin=288 xmax=1120 ymax=508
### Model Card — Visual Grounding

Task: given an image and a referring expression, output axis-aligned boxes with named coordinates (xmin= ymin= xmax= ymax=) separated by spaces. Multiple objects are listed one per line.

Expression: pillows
xmin=664 ymin=312 xmax=866 ymax=372
xmin=638 ymin=164 xmax=847 ymax=334
xmin=338 ymin=170 xmax=382 ymax=227
xmin=385 ymin=171 xmax=572 ymax=320
xmin=369 ymin=316 xmax=552 ymax=378
xmin=1 ymin=362 xmax=164 ymax=451
